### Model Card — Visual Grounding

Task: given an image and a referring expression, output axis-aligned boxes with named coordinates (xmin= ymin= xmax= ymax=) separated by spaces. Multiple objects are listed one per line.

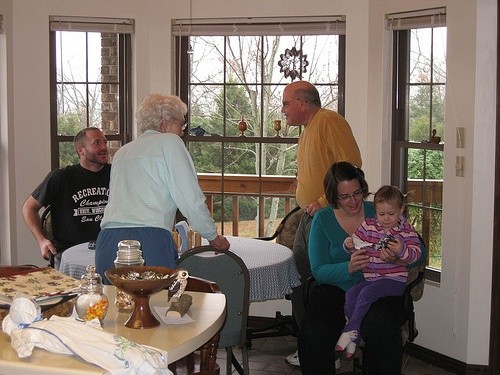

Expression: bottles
xmin=75 ymin=265 xmax=109 ymax=326
xmin=114 ymin=240 xmax=145 ymax=314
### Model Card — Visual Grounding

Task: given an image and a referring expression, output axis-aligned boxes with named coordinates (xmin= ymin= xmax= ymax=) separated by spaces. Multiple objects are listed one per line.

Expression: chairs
xmin=175 ymin=245 xmax=251 ymax=375
xmin=351 ymin=231 xmax=427 ymax=375
xmin=40 ymin=204 xmax=58 ymax=269
xmin=240 ymin=205 xmax=309 ymax=351
xmin=168 ymin=276 xmax=226 ymax=374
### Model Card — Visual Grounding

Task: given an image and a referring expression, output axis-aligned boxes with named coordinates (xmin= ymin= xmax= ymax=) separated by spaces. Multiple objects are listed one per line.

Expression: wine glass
xmin=273 ymin=120 xmax=282 ymax=138
xmin=104 ymin=265 xmax=179 ymax=329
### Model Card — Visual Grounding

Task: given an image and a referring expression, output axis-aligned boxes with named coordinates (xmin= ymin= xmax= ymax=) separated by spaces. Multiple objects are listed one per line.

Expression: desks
xmin=60 ymin=231 xmax=301 ymax=375
xmin=0 ymin=282 xmax=227 ymax=375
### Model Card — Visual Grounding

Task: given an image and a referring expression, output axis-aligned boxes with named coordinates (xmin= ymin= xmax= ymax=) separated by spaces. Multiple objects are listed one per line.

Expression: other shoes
xmin=286 ymin=347 xmax=302 ymax=366
xmin=335 ymin=357 xmax=341 ymax=369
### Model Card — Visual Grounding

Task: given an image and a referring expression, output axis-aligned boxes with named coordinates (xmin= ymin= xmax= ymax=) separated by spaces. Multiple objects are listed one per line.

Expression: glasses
xmin=336 ymin=189 xmax=364 ymax=201
xmin=174 ymin=117 xmax=188 ymax=130
xmin=283 ymin=97 xmax=308 ymax=107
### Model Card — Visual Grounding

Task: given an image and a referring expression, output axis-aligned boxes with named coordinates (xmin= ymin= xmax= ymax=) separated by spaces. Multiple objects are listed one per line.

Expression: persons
xmin=295 ymin=161 xmax=427 ymax=375
xmin=94 ymin=94 xmax=230 ymax=286
xmin=21 ymin=127 xmax=113 ymax=271
xmin=281 ymin=80 xmax=363 ymax=371
xmin=334 ymin=184 xmax=423 ymax=359
